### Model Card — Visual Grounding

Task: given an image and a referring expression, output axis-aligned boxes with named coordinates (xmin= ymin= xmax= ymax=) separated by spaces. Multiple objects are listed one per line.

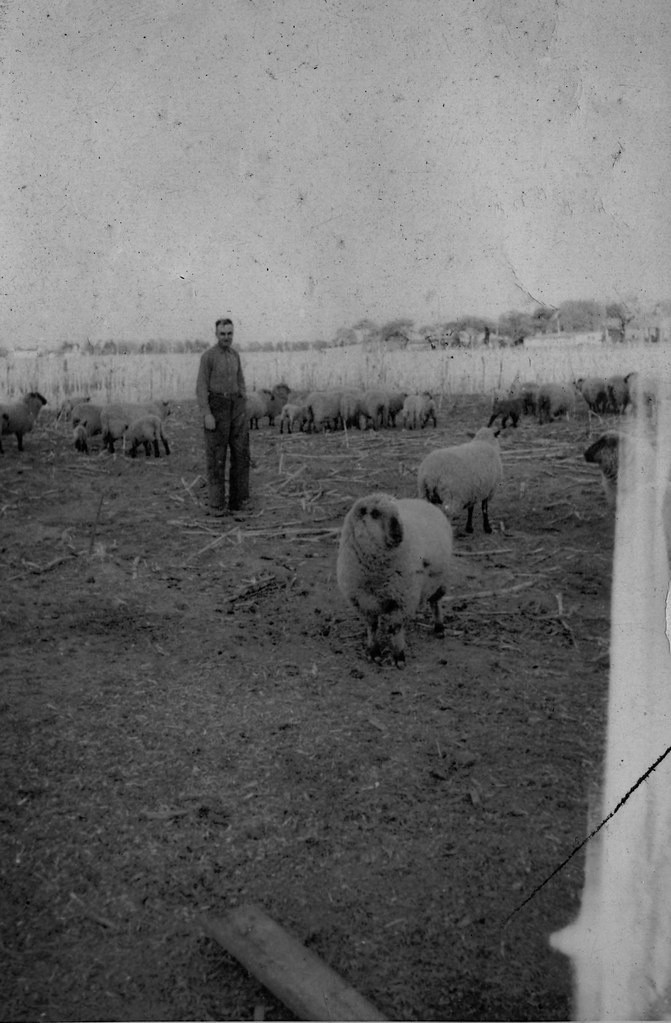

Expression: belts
xmin=207 ymin=390 xmax=238 ymax=398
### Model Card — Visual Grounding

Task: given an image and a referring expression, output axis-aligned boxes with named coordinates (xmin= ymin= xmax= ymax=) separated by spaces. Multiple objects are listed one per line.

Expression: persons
xmin=483 ymin=327 xmax=490 ymax=344
xmin=197 ymin=319 xmax=251 ymax=515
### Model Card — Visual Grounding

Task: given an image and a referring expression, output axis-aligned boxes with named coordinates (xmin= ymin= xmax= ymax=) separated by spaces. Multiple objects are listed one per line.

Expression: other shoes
xmin=227 ymin=504 xmax=254 ymax=515
xmin=207 ymin=504 xmax=224 ymax=516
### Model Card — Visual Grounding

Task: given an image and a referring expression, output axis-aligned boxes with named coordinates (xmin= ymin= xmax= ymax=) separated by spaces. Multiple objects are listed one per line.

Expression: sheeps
xmin=335 ymin=491 xmax=455 ymax=670
xmin=247 ymin=383 xmax=438 ymax=435
xmin=415 ymin=426 xmax=504 ymax=535
xmin=582 ymin=429 xmax=618 ymax=512
xmin=486 ymin=368 xmax=665 ymax=430
xmin=0 ymin=391 xmax=48 ymax=456
xmin=56 ymin=397 xmax=172 ymax=458
